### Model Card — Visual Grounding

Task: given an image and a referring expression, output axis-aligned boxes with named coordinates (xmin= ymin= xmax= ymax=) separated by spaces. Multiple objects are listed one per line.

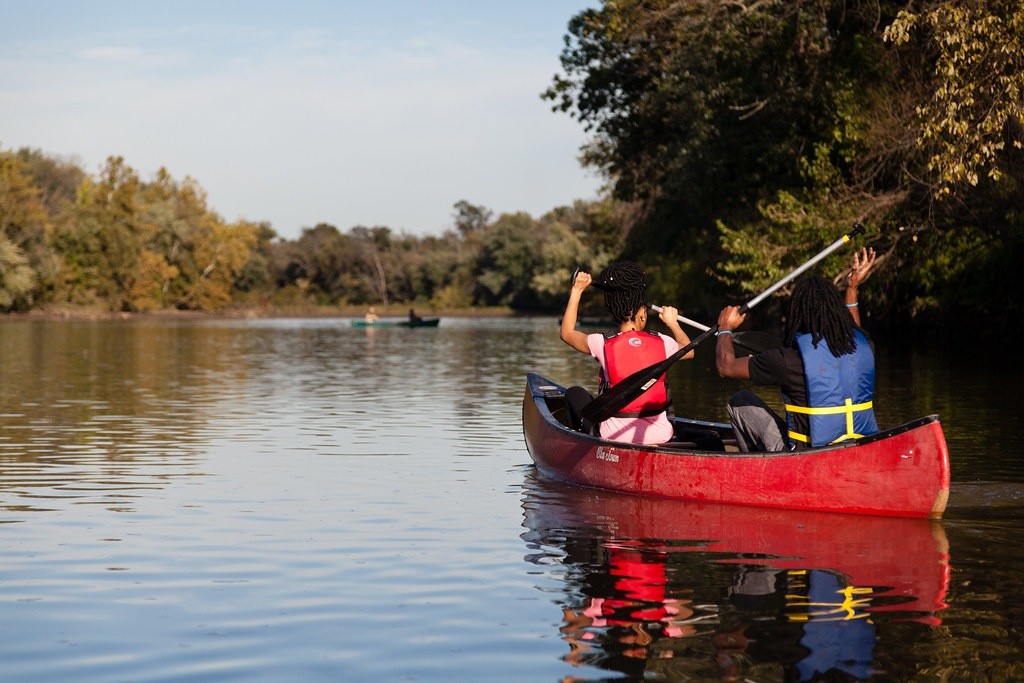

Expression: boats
xmin=521 ymin=371 xmax=951 ymax=520
xmin=351 ymin=317 xmax=439 ymax=328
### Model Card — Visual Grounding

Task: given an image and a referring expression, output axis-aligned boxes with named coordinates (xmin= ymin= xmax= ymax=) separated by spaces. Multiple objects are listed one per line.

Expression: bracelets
xmin=717 ymin=331 xmax=734 ymax=338
xmin=845 ymin=302 xmax=858 ymax=307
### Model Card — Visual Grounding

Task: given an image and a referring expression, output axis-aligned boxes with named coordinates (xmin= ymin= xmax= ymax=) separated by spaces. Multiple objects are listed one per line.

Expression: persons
xmin=560 ymin=262 xmax=695 ymax=445
xmin=365 ymin=307 xmax=378 ymax=323
xmin=716 ymin=247 xmax=878 ymax=453
xmin=409 ymin=309 xmax=422 ymax=321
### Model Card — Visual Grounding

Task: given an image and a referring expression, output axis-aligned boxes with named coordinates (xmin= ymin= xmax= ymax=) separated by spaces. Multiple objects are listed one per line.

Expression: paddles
xmin=581 ymin=220 xmax=867 ymax=429
xmin=572 ymin=266 xmax=772 ymax=357
